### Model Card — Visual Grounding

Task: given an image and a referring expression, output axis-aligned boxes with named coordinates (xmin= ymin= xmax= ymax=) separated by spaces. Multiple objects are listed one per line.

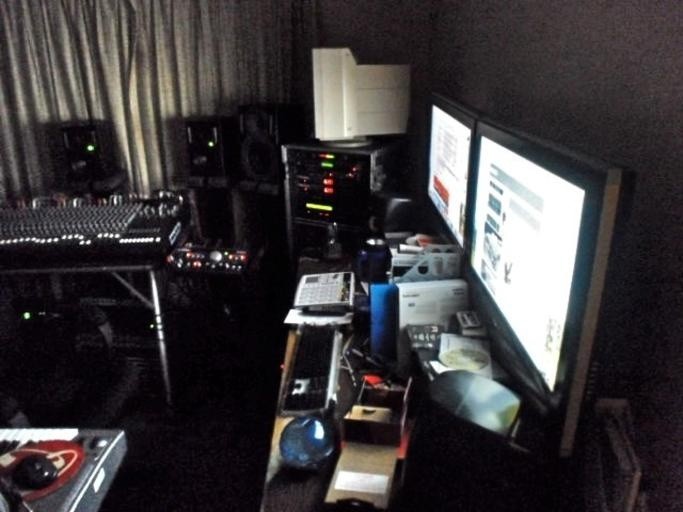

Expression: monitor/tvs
xmin=463 ymin=119 xmax=637 ymax=463
xmin=310 ymin=43 xmax=412 ymax=147
xmin=419 ymin=91 xmax=482 ymax=256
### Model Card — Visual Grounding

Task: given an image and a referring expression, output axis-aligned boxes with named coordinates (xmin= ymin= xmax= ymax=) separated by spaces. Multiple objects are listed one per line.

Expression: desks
xmin=257 ymin=253 xmax=584 ymax=512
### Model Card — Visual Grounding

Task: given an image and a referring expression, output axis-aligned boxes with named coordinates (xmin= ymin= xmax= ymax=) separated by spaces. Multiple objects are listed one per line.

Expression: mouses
xmin=13 ymin=454 xmax=59 ymax=487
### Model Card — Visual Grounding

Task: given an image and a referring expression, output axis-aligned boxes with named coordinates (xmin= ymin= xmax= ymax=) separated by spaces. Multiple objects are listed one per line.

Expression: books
xmin=563 ymin=441 xmax=610 ymax=512
xmin=322 ymin=438 xmax=398 ymax=510
xmin=595 ymin=409 xmax=642 ymax=511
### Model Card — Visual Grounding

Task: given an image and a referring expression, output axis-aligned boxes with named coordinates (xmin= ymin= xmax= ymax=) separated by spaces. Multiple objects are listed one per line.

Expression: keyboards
xmin=278 ymin=321 xmax=339 ymax=420
xmin=293 ymin=271 xmax=355 ymax=306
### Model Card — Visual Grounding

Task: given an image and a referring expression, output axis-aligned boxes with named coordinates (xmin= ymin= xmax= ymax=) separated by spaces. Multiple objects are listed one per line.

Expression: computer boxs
xmin=185 ymin=114 xmax=236 ymax=188
xmin=60 ymin=119 xmax=118 ymax=175
xmin=238 ymin=104 xmax=307 ymax=195
